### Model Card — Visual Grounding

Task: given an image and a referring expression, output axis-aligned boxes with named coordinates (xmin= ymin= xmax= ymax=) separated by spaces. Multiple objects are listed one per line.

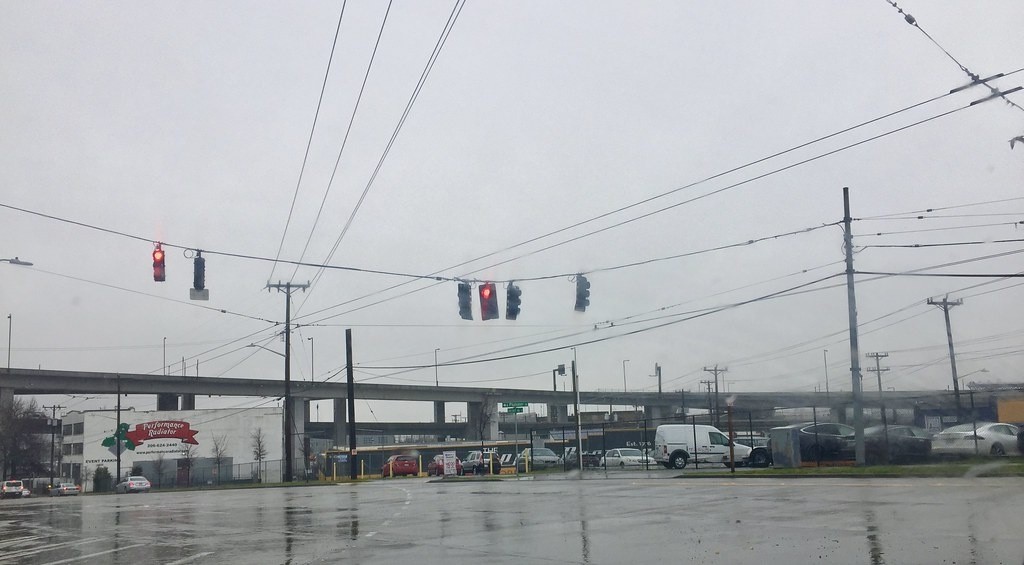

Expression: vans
xmin=654 ymin=425 xmax=752 ymax=469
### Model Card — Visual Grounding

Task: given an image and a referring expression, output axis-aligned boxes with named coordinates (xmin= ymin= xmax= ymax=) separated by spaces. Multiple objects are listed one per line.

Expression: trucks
xmin=726 ymin=419 xmax=1024 ymax=468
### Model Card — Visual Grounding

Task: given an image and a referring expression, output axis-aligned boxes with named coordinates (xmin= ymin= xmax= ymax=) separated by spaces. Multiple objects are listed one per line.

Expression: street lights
xmin=953 ymin=369 xmax=991 ymax=424
xmin=623 ymin=359 xmax=629 ymax=392
xmin=434 ymin=348 xmax=440 ymax=386
xmin=8 ymin=313 xmax=11 ymax=368
xmin=823 ymin=349 xmax=828 ymax=392
xmin=247 ymin=342 xmax=293 ymax=483
xmin=571 ymin=347 xmax=576 ymax=392
xmin=308 ymin=337 xmax=313 ymax=382
xmin=163 ymin=337 xmax=166 ymax=374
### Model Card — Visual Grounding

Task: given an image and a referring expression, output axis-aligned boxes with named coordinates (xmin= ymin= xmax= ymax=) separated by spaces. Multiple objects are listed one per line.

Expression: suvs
xmin=462 ymin=449 xmax=501 ymax=475
xmin=0 ymin=480 xmax=24 ymax=499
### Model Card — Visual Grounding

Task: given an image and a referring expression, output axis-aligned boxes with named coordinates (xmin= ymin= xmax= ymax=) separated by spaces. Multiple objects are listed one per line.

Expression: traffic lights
xmin=478 ymin=283 xmax=499 ymax=321
xmin=457 ymin=283 xmax=472 ymax=320
xmin=194 ymin=257 xmax=206 ymax=290
xmin=152 ymin=250 xmax=166 ymax=282
xmin=574 ymin=275 xmax=590 ymax=312
xmin=506 ymin=286 xmax=522 ymax=321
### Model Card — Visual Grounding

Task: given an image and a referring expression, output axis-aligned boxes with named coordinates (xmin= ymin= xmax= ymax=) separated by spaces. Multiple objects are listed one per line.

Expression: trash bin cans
xmin=518 ymin=457 xmax=526 ymax=473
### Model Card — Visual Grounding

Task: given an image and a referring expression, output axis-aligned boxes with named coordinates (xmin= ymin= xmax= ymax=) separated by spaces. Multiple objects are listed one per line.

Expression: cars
xmin=115 ymin=476 xmax=152 ymax=494
xmin=48 ymin=483 xmax=81 ymax=497
xmin=22 ymin=489 xmax=31 ymax=498
xmin=380 ymin=455 xmax=418 ymax=477
xmin=428 ymin=454 xmax=461 ymax=476
xmin=517 ymin=447 xmax=657 ymax=472
